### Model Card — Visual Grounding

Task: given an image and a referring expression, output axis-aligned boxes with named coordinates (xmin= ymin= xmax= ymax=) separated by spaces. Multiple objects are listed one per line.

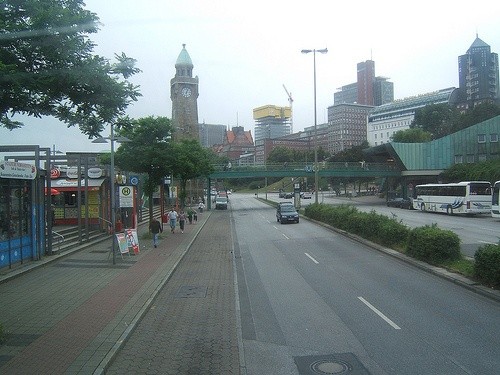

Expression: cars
xmin=386 ymin=198 xmax=413 ymax=210
xmin=301 ymin=48 xmax=328 ymax=203
xmin=279 ymin=192 xmax=312 ymax=199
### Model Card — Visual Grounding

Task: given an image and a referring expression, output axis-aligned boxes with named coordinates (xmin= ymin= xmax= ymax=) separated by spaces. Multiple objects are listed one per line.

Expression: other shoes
xmin=182 ymin=231 xmax=183 ymax=233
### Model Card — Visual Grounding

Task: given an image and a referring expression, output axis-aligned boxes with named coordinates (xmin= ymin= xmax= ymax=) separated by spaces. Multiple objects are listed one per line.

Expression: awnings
xmin=45 ymin=177 xmax=106 ymax=192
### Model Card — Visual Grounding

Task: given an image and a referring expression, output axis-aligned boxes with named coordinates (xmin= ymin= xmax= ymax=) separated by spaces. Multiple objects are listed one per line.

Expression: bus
xmin=412 ymin=181 xmax=500 ymax=219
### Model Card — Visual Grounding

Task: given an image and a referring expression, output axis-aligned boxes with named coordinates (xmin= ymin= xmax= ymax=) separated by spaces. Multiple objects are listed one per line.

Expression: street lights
xmin=91 ymin=135 xmax=133 ymax=264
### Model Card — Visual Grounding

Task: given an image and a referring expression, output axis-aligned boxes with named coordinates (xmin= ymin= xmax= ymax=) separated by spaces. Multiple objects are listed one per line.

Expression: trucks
xmin=216 ymin=191 xmax=228 ymax=209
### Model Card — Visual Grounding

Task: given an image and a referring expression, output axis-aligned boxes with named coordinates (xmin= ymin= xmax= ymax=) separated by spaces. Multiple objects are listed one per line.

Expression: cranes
xmin=283 ymin=84 xmax=294 ymax=134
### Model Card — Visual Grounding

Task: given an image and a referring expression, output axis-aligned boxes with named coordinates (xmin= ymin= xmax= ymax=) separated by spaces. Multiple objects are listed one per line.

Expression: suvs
xmin=276 ymin=203 xmax=299 ymax=224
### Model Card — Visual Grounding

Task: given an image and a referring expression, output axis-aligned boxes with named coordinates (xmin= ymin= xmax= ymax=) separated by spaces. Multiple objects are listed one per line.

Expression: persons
xmin=199 ymin=201 xmax=205 ymax=214
xmin=149 ymin=217 xmax=164 ymax=248
xmin=226 ymin=160 xmax=233 ymax=169
xmin=179 ymin=211 xmax=188 ymax=234
xmin=187 ymin=209 xmax=197 ymax=225
xmin=223 ymin=164 xmax=226 ymax=171
xmin=168 ymin=208 xmax=179 ymax=234
xmin=321 ymin=160 xmax=325 ymax=170
xmin=372 ymin=186 xmax=375 ymax=191
xmin=345 ymin=162 xmax=348 ymax=170
xmin=122 ymin=210 xmax=127 ymax=229
xmin=359 ymin=159 xmax=368 ymax=171
xmin=336 ymin=189 xmax=341 ymax=195
xmin=284 ymin=161 xmax=289 ymax=168
